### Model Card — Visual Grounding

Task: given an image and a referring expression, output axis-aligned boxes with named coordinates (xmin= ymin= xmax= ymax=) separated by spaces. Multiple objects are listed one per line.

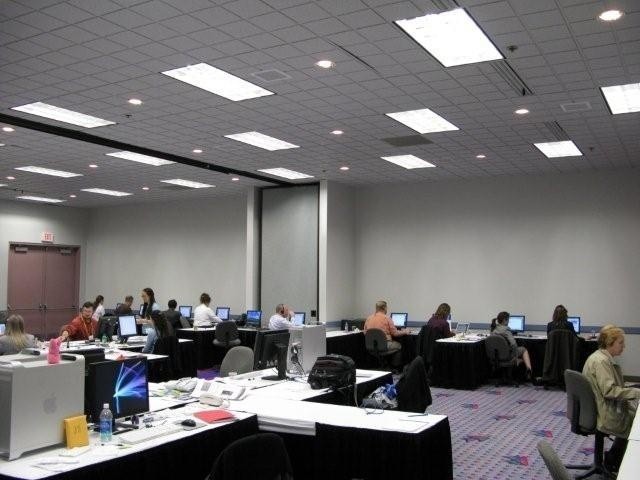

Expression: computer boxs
xmin=0 ymin=348 xmax=84 ymax=462
xmin=288 ymin=325 xmax=326 ymax=375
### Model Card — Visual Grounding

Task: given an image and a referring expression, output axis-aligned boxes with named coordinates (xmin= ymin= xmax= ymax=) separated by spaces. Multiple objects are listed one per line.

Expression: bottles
xmin=100 ymin=404 xmax=112 ymax=443
xmin=345 ymin=321 xmax=348 ymax=334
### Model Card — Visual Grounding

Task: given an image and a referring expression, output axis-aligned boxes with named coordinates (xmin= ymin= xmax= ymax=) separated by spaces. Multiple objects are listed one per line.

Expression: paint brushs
xmin=407 ymin=413 xmax=429 ymax=417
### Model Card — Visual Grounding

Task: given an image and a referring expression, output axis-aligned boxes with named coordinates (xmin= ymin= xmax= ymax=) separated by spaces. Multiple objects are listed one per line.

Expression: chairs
xmin=418 ymin=326 xmax=441 ymax=378
xmin=154 ymin=338 xmax=181 ymax=381
xmin=360 ymin=356 xmax=432 ymax=412
xmin=485 ymin=335 xmax=522 ymax=387
xmin=365 ymin=329 xmax=399 ymax=369
xmin=207 ymin=433 xmax=293 ymax=480
xmin=213 ymin=321 xmax=241 ymax=369
xmin=565 ymin=368 xmax=618 ymax=480
xmin=545 ymin=329 xmax=578 ymax=390
xmin=537 ymin=441 xmax=571 ymax=480
xmin=221 ymin=347 xmax=254 ymax=376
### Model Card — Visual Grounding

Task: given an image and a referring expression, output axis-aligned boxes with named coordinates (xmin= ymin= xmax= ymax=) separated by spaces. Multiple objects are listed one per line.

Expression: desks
xmin=178 ymin=327 xmax=258 ymax=370
xmin=326 ymin=330 xmax=365 ymax=360
xmin=257 ymin=402 xmax=450 ymax=480
xmin=148 ymin=368 xmax=286 ymax=410
xmin=513 ymin=331 xmax=603 ymax=379
xmin=0 ymin=402 xmax=257 ymax=480
xmin=435 ymin=329 xmax=490 ymax=391
xmin=245 ymin=370 xmax=393 ymax=401
xmin=402 ymin=327 xmax=422 ymax=369
xmin=618 ymin=404 xmax=640 ymax=480
xmin=44 ymin=335 xmax=195 ymax=376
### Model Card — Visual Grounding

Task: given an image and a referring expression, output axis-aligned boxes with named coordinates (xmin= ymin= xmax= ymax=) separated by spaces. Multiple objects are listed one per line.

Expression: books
xmin=190 ymin=377 xmax=259 ymax=401
xmin=193 ymin=409 xmax=236 ymax=423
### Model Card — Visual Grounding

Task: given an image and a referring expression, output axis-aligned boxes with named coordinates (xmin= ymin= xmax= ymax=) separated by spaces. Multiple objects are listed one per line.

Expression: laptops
xmin=454 ymin=323 xmax=469 ymax=335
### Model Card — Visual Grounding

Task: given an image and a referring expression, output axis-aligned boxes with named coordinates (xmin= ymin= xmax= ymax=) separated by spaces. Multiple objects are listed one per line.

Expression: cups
xmin=228 ymin=371 xmax=238 ymax=383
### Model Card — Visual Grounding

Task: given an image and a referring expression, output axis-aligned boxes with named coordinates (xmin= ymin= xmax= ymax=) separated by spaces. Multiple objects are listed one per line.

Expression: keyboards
xmin=119 ymin=423 xmax=180 ymax=446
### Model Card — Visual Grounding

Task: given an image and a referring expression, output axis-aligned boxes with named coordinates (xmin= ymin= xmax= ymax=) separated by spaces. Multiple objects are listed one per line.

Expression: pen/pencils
xmin=101 ymin=444 xmax=123 ymax=446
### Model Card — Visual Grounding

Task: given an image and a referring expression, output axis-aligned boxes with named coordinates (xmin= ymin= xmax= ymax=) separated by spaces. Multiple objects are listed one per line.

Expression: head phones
xmin=281 ymin=304 xmax=284 ymax=314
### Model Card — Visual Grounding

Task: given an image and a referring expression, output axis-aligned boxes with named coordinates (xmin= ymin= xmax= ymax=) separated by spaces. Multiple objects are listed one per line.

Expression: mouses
xmin=181 ymin=419 xmax=196 ymax=427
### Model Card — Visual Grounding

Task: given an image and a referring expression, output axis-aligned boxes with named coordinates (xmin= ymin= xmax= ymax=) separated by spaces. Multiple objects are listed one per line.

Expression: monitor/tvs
xmin=140 ymin=305 xmax=144 ymax=315
xmin=289 ymin=313 xmax=305 ymax=324
xmin=247 ymin=310 xmax=261 ymax=325
xmin=0 ymin=324 xmax=5 ymax=336
xmin=216 ymin=307 xmax=230 ymax=320
xmin=566 ymin=317 xmax=580 ymax=334
xmin=95 ymin=317 xmax=117 ymax=342
xmin=179 ymin=306 xmax=192 ymax=318
xmin=508 ymin=316 xmax=525 ymax=332
xmin=255 ymin=329 xmax=290 ymax=380
xmin=61 ymin=348 xmax=105 ymax=414
xmin=391 ymin=313 xmax=407 ymax=330
xmin=118 ymin=314 xmax=138 ymax=344
xmin=89 ymin=356 xmax=149 ymax=435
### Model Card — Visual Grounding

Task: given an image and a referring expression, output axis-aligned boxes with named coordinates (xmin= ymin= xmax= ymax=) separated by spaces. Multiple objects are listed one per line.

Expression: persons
xmin=547 ymin=305 xmax=592 ymax=343
xmin=0 ymin=314 xmax=43 ymax=356
xmin=364 ymin=301 xmax=407 ymax=372
xmin=583 ymin=324 xmax=640 ymax=472
xmin=142 ymin=309 xmax=175 ymax=354
xmin=163 ymin=299 xmax=191 ymax=328
xmin=57 ymin=302 xmax=98 ymax=342
xmin=491 ymin=312 xmax=532 ymax=379
xmin=193 ymin=293 xmax=223 ymax=328
xmin=115 ymin=296 xmax=136 ymax=316
xmin=268 ymin=304 xmax=295 ymax=331
xmin=92 ymin=296 xmax=106 ymax=322
xmin=426 ymin=303 xmax=456 ymax=338
xmin=0 ymin=310 xmax=7 ymax=333
xmin=136 ymin=288 xmax=161 ymax=336
